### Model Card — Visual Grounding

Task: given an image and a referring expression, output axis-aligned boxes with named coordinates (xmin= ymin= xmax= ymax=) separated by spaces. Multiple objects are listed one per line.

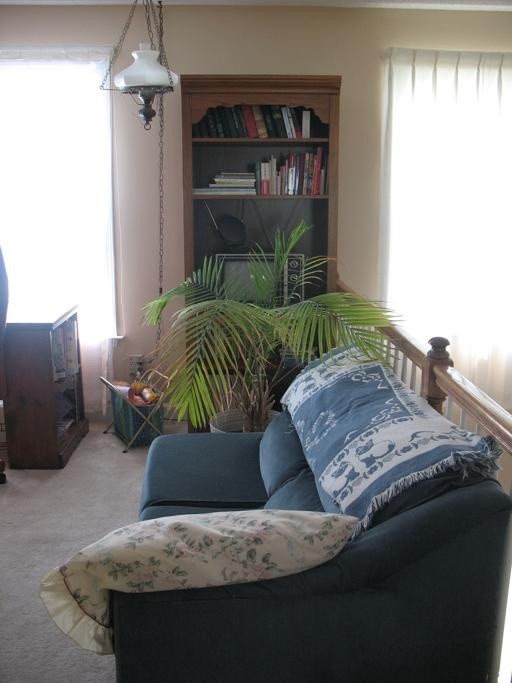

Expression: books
xmin=50 ymin=319 xmax=79 ymax=380
xmin=57 ymin=418 xmax=76 ymax=454
xmin=192 ymin=103 xmax=328 ymax=197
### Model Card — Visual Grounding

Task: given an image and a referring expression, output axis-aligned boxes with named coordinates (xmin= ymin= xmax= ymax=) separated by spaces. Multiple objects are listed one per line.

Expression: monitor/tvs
xmin=215 ymin=253 xmax=304 ymax=309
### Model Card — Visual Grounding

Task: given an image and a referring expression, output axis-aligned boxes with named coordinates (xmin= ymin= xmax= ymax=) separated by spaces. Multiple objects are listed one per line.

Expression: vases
xmin=138 ymin=217 xmax=405 ymax=433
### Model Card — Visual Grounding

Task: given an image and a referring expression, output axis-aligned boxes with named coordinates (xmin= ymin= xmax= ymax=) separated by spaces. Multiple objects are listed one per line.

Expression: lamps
xmin=100 ymin=0 xmax=181 ymax=129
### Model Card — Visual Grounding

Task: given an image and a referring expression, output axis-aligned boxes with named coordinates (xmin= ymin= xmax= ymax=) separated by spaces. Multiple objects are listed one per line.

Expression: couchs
xmin=110 ymin=341 xmax=510 ymax=683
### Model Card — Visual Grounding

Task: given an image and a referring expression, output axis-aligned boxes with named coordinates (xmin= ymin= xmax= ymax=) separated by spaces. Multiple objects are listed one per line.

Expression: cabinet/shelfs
xmin=0 ymin=305 xmax=89 ymax=469
xmin=178 ymin=72 xmax=342 ymax=432
